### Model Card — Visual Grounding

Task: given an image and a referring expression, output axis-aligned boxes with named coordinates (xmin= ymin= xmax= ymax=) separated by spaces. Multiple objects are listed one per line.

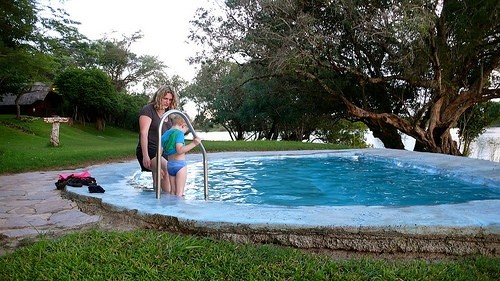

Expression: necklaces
xmin=158 ymin=110 xmax=162 ymax=117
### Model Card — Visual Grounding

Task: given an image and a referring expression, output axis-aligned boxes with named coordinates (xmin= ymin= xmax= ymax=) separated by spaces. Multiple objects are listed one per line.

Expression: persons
xmin=136 ymin=85 xmax=192 ymax=194
xmin=161 ymin=112 xmax=202 ymax=199
